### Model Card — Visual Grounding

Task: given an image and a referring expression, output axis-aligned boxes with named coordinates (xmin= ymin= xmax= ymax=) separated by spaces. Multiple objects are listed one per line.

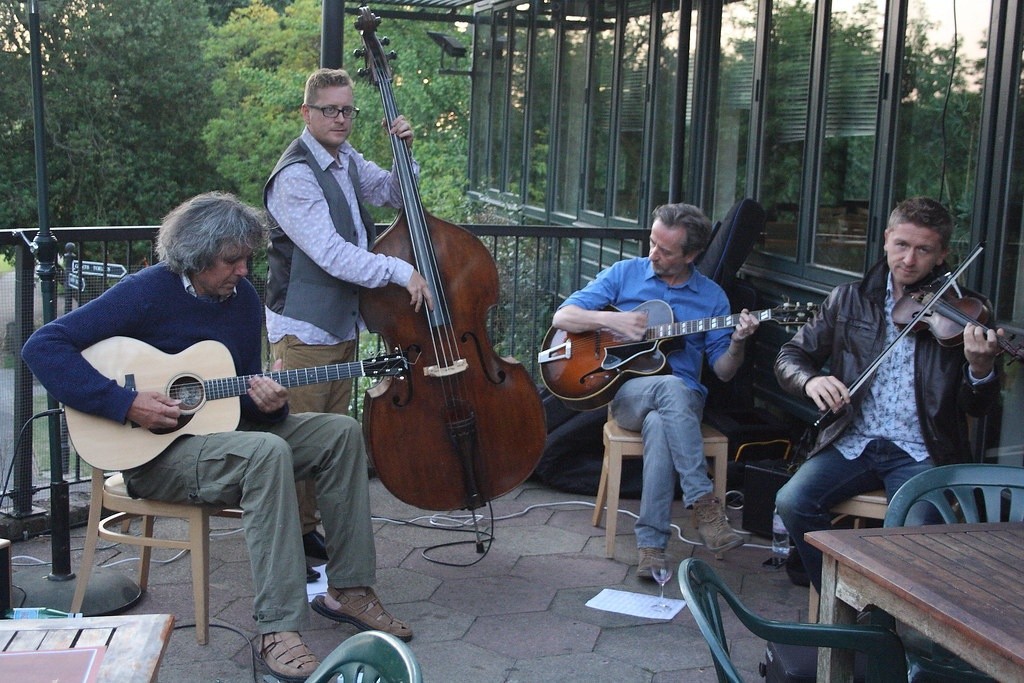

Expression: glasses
xmin=307 ymin=104 xmax=360 ymax=119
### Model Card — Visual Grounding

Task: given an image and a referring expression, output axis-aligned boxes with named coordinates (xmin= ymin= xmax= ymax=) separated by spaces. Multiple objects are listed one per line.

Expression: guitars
xmin=64 ymin=335 xmax=411 ymax=471
xmin=537 ymin=298 xmax=821 ymax=413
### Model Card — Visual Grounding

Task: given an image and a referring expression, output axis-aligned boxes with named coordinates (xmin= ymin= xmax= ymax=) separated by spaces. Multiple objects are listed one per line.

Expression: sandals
xmin=254 ymin=631 xmax=322 ymax=683
xmin=311 ymin=586 xmax=413 ymax=642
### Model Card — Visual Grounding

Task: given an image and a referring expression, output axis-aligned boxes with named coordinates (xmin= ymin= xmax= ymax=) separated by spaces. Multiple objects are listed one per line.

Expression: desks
xmin=0 ymin=613 xmax=176 ymax=683
xmin=803 ymin=522 xmax=1024 ymax=683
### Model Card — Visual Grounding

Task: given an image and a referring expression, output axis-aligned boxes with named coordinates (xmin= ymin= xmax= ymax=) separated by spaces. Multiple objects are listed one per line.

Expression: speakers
xmin=742 ymin=460 xmax=801 ymax=538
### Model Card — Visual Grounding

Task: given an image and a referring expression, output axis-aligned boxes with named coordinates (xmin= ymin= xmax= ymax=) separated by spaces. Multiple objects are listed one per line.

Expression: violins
xmin=890 ymin=273 xmax=1024 ymax=367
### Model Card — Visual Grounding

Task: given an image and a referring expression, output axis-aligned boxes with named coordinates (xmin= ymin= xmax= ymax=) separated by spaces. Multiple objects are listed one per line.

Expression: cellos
xmin=353 ymin=6 xmax=548 ymax=553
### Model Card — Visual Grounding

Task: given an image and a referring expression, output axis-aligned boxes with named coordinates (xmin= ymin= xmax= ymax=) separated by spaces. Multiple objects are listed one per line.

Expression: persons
xmin=20 ymin=189 xmax=412 ymax=681
xmin=775 ymin=195 xmax=1001 ymax=677
xmin=550 ymin=202 xmax=760 ymax=582
xmin=262 ymin=68 xmax=420 ymax=581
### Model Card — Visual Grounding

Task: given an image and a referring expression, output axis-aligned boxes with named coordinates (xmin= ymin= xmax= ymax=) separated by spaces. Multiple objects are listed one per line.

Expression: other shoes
xmin=302 ymin=529 xmax=329 ymax=560
xmin=692 ymin=492 xmax=744 ymax=553
xmin=636 ymin=547 xmax=667 ymax=579
xmin=306 ymin=561 xmax=320 ymax=582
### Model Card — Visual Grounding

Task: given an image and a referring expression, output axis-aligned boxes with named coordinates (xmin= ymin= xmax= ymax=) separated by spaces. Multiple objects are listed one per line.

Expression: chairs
xmin=808 ymin=486 xmax=890 ymax=625
xmin=302 ymin=630 xmax=424 ymax=683
xmin=883 ymin=462 xmax=1024 ymax=528
xmin=69 ymin=464 xmax=245 ymax=646
xmin=676 ymin=555 xmax=914 ymax=683
xmin=584 ymin=400 xmax=736 ymax=571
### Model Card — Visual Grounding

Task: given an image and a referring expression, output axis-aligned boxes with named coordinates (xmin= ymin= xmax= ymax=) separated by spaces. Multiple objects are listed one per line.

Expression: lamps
xmin=427 ymin=28 xmax=472 ymax=79
